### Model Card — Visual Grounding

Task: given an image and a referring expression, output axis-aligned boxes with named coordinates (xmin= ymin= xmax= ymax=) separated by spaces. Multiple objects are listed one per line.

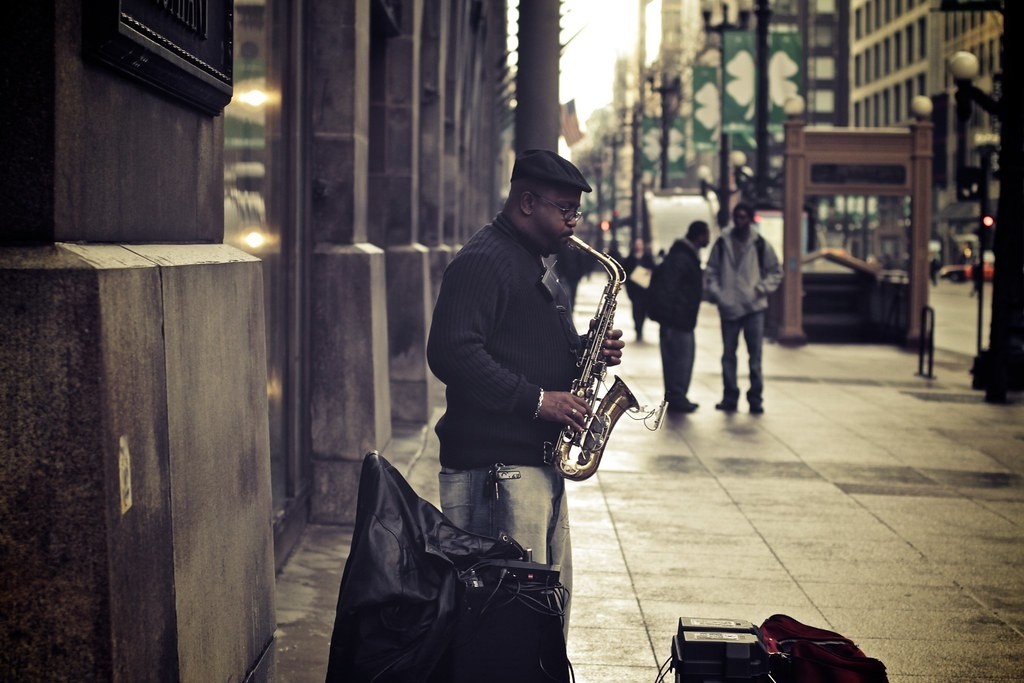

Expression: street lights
xmin=973 ymin=131 xmax=1001 ymax=353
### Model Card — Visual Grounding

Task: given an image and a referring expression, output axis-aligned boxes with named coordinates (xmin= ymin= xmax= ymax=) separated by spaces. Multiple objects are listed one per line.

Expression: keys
xmin=489 ymin=475 xmax=500 ymax=500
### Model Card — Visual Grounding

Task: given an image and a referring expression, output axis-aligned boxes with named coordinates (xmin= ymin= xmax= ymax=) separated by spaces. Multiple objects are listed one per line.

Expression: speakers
xmin=453 ymin=583 xmax=570 ymax=683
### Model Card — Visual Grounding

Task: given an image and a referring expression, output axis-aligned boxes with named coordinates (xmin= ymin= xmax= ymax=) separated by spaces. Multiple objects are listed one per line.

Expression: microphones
xmin=653 ymin=393 xmax=673 ymax=431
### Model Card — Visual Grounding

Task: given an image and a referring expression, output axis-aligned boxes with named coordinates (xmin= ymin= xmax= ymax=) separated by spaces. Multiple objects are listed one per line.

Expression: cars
xmin=938 ymin=263 xmax=996 ymax=285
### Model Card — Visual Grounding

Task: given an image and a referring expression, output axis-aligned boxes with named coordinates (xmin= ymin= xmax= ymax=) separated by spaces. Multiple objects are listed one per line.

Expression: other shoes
xmin=668 ymin=397 xmax=698 ymax=413
xmin=749 ymin=402 xmax=763 ymax=414
xmin=715 ymin=400 xmax=737 ymax=411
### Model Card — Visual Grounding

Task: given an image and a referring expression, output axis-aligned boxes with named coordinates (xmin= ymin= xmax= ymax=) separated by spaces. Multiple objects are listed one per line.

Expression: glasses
xmin=529 ymin=186 xmax=583 ymax=222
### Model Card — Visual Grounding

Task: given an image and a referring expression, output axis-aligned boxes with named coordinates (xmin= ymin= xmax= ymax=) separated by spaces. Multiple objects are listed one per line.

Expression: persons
xmin=561 ymin=201 xmax=980 ymax=415
xmin=427 ymin=150 xmax=624 ymax=644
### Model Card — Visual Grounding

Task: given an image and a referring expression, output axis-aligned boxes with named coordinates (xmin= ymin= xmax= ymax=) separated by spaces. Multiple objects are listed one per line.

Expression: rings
xmin=571 ymin=408 xmax=577 ymax=417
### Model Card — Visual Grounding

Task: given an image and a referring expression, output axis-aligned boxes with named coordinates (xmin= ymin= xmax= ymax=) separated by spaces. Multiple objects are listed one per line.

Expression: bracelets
xmin=530 ymin=388 xmax=544 ymax=425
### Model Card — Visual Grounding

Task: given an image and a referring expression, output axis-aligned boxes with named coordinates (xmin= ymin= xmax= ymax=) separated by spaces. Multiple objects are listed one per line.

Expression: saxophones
xmin=556 ymin=234 xmax=670 ymax=481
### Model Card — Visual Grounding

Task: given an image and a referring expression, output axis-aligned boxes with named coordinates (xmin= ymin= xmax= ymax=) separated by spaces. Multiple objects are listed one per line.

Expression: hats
xmin=509 ymin=149 xmax=595 ymax=194
